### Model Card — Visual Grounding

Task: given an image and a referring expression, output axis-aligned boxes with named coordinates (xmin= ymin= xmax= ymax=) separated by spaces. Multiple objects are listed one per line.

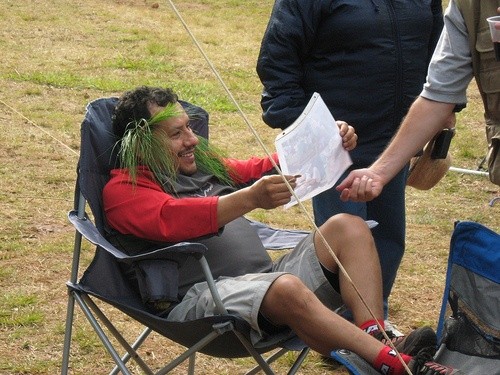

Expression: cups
xmin=486 ymin=16 xmax=500 ymax=62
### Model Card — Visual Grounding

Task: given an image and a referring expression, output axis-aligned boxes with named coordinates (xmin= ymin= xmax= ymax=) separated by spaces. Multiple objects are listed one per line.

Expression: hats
xmin=404 ymin=130 xmax=455 ymax=190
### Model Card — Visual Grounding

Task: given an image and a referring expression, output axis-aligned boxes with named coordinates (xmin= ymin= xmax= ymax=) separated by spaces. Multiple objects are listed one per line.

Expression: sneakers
xmin=410 ymin=347 xmax=463 ymax=375
xmin=377 ymin=326 xmax=436 ymax=359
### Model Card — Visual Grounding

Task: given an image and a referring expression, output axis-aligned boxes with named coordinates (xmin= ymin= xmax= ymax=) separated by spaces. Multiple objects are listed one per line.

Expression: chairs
xmin=330 ymin=219 xmax=500 ymax=375
xmin=61 ymin=95 xmax=380 ymax=375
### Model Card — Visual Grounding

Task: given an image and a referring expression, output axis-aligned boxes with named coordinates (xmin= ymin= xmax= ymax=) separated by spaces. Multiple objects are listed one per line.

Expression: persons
xmin=256 ymin=1 xmax=467 ymax=325
xmin=335 ymin=0 xmax=500 ymax=203
xmin=101 ymin=85 xmax=462 ymax=375
